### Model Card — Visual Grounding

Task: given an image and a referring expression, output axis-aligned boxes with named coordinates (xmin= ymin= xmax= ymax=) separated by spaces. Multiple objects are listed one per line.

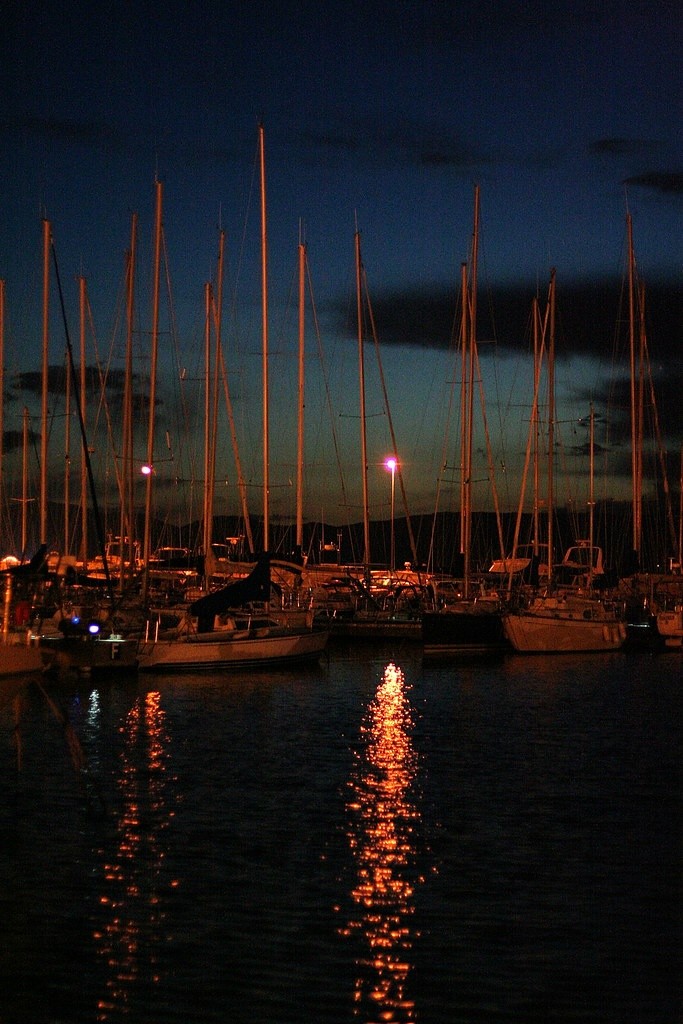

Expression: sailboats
xmin=0 ymin=125 xmax=683 ymax=678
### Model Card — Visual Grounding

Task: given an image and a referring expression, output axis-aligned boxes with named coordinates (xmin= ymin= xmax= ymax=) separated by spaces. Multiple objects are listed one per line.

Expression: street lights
xmin=386 ymin=459 xmax=398 ymax=569
